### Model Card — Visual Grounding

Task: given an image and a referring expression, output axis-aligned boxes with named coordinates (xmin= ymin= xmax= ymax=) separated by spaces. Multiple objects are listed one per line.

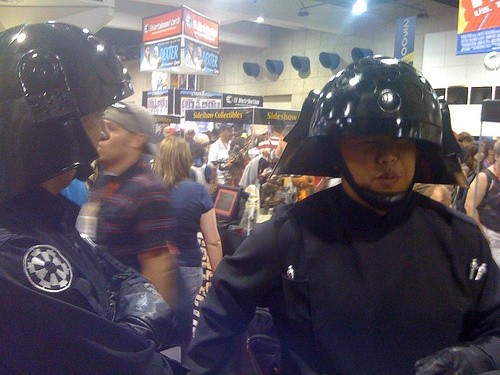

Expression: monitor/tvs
xmin=211 ymin=189 xmax=238 ymax=216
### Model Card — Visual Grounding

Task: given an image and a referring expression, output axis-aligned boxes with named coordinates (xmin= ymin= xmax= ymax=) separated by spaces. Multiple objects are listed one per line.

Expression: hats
xmin=103 ymin=98 xmax=157 ymax=157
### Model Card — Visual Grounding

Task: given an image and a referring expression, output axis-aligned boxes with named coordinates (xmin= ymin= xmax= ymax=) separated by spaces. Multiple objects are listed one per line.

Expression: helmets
xmin=0 ymin=20 xmax=137 ymax=202
xmin=268 ymin=57 xmax=469 ymax=188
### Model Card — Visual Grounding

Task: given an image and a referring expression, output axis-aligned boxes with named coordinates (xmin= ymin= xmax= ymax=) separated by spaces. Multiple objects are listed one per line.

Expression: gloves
xmin=408 ymin=336 xmax=500 ymax=375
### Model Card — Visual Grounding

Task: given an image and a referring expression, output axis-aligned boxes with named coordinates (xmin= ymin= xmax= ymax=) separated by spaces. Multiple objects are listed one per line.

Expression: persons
xmin=77 ymin=100 xmax=180 ymax=310
xmin=141 ymin=45 xmax=158 ymax=71
xmin=414 ymin=132 xmax=500 ymax=266
xmin=187 ymin=55 xmax=500 ymax=375
xmin=153 ymin=137 xmax=223 ymax=301
xmin=158 ymin=120 xmax=341 ymax=232
xmin=0 ymin=21 xmax=192 ymax=375
xmin=185 ymin=42 xmax=205 ymax=71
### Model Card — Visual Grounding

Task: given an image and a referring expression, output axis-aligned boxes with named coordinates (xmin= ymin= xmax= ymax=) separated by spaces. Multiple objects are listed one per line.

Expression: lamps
xmin=298 ymin=3 xmax=326 ymax=16
xmin=405 ymin=5 xmax=429 ymax=17
